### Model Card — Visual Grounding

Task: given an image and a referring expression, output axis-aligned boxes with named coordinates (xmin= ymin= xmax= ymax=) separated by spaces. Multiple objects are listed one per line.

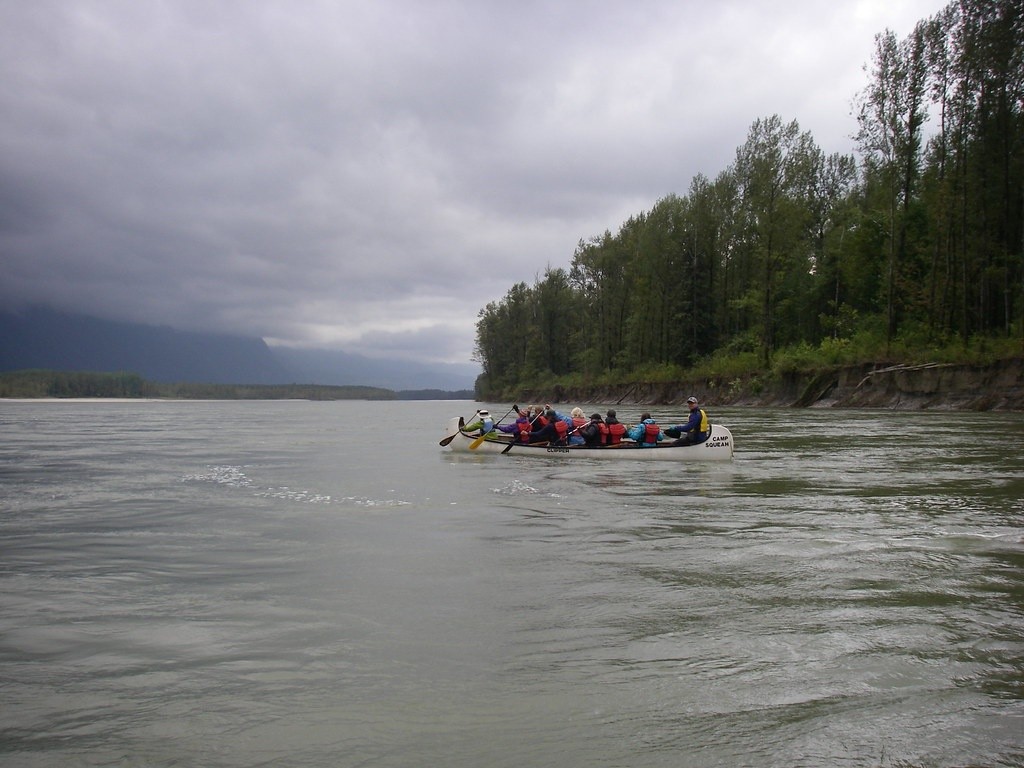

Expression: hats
xmin=687 ymin=396 xmax=698 ymax=403
xmin=589 ymin=413 xmax=601 ymax=418
xmin=520 ymin=408 xmax=529 ymax=416
xmin=477 ymin=409 xmax=491 ymax=418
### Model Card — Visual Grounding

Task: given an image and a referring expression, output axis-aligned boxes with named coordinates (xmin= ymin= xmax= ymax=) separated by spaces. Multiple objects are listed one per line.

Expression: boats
xmin=447 ymin=415 xmax=734 ymax=463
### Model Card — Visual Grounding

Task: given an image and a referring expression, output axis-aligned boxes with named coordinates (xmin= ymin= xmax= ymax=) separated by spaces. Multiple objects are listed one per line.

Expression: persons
xmin=460 ymin=409 xmax=498 ymax=440
xmin=626 ymin=412 xmax=663 ymax=447
xmin=670 ymin=397 xmax=707 ymax=446
xmin=546 ymin=404 xmax=589 ymax=445
xmin=604 ymin=409 xmax=629 ymax=444
xmin=521 ymin=410 xmax=568 ymax=446
xmin=577 ymin=413 xmax=610 ymax=447
xmin=492 ymin=405 xmax=533 ymax=443
xmin=527 ymin=405 xmax=548 ymax=432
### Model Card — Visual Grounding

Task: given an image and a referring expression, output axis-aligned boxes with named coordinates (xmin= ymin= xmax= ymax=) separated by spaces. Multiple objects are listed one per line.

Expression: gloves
xmin=493 ymin=425 xmax=498 ymax=429
xmin=513 ymin=405 xmax=519 ymax=412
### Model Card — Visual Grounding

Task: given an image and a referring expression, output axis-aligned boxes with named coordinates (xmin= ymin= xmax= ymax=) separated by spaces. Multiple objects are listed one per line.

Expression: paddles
xmin=565 ymin=420 xmax=591 ymax=441
xmin=469 ymin=406 xmax=513 ymax=449
xmin=500 ymin=406 xmax=549 ymax=455
xmin=438 ymin=409 xmax=482 ymax=446
